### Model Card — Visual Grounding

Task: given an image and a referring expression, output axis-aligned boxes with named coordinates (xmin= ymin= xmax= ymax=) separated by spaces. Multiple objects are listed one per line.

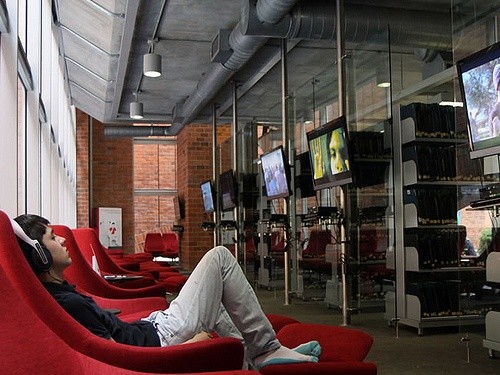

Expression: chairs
xmin=46 ymin=224 xmax=168 ymax=317
xmin=71 ymin=228 xmax=189 ymax=292
xmin=245 ymin=228 xmax=385 ymax=258
xmin=0 ymin=210 xmax=378 ymax=375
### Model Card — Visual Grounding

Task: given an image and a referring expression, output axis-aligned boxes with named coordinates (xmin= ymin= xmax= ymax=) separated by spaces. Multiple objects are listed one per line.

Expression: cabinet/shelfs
xmin=382 ymin=103 xmax=486 ymax=336
xmin=254 ymin=153 xmax=288 ymax=291
xmin=323 ymin=131 xmax=389 ymax=314
xmin=289 ymin=146 xmax=326 ymax=302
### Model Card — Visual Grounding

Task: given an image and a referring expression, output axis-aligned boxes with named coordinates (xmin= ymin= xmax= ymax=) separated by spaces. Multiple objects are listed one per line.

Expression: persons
xmin=312 ymin=127 xmax=350 ymax=179
xmin=13 ymin=214 xmax=321 ymax=368
xmin=490 ymin=64 xmax=500 ymax=137
xmin=263 ymin=164 xmax=287 ymax=197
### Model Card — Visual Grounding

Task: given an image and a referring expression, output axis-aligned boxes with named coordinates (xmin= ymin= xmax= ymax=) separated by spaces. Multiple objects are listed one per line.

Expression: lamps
xmin=144 ymin=39 xmax=161 ymax=77
xmin=376 ymin=67 xmax=389 ymax=86
xmin=128 ymin=92 xmax=144 ymax=119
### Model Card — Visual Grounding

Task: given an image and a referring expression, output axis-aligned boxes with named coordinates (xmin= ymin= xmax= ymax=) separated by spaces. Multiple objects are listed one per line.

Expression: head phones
xmin=10 ymin=218 xmax=54 ymax=271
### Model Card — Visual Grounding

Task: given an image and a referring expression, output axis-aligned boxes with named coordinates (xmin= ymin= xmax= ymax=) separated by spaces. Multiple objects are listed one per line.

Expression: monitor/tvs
xmin=219 ymin=169 xmax=237 ymax=212
xmin=456 ymin=41 xmax=500 ymax=160
xmin=259 ymin=145 xmax=290 ymax=201
xmin=173 ymin=196 xmax=182 ymax=221
xmin=306 ymin=114 xmax=354 ymax=191
xmin=199 ymin=180 xmax=216 ymax=213
